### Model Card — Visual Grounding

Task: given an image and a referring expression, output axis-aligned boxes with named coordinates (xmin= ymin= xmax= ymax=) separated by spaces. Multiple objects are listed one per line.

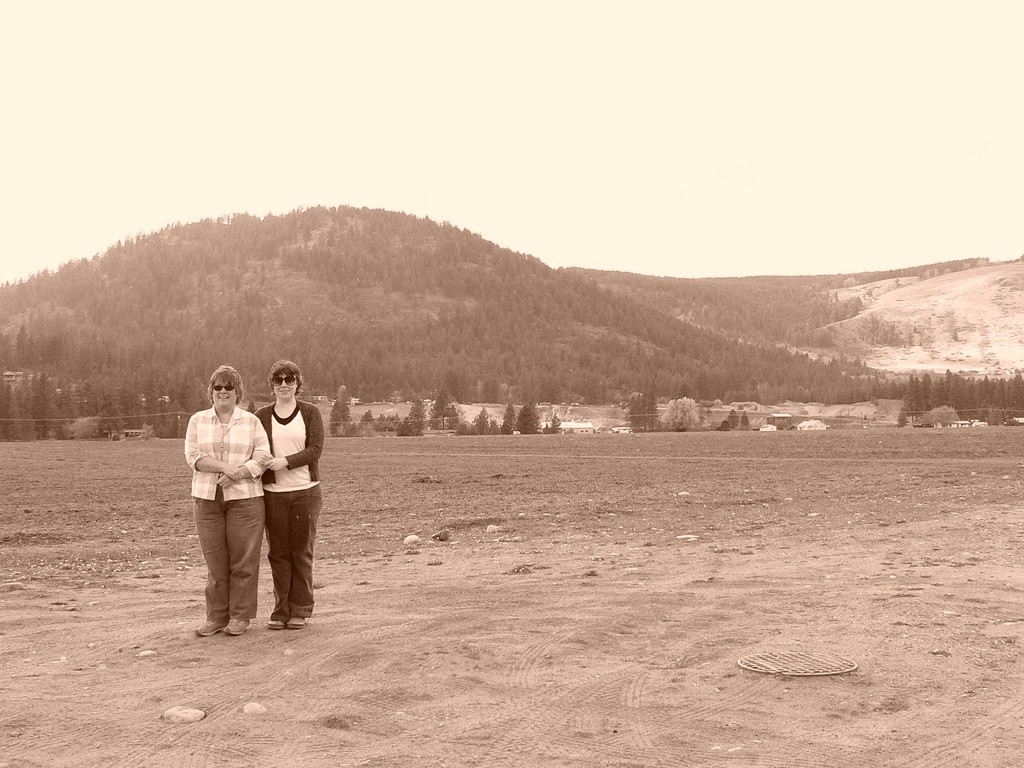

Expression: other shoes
xmin=287 ymin=617 xmax=305 ymax=628
xmin=268 ymin=619 xmax=286 ymax=629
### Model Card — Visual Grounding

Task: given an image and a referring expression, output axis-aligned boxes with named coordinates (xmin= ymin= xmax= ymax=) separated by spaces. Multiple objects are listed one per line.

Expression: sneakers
xmin=223 ymin=618 xmax=250 ymax=635
xmin=195 ymin=621 xmax=229 ymax=636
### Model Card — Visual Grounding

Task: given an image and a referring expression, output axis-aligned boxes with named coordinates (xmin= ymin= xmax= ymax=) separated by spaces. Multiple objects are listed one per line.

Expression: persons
xmin=185 ymin=366 xmax=270 ymax=635
xmin=252 ymin=360 xmax=323 ymax=630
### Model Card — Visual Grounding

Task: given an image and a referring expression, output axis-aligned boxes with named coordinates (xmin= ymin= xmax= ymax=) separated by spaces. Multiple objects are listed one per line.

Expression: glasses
xmin=271 ymin=376 xmax=296 ymax=385
xmin=212 ymin=385 xmax=235 ymax=391
